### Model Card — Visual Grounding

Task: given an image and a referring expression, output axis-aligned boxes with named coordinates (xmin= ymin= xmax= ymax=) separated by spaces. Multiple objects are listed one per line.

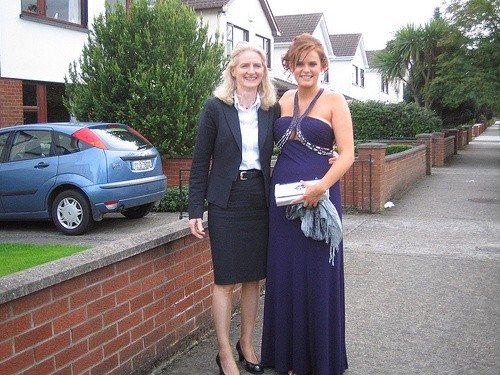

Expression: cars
xmin=0 ymin=122 xmax=168 ymax=236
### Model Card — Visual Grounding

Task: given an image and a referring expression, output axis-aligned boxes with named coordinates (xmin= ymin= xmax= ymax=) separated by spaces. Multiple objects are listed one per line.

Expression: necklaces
xmin=244 ymin=98 xmax=256 ymax=110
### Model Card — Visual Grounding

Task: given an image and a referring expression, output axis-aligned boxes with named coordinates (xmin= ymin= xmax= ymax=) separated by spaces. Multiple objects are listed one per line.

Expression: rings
xmin=311 ymin=200 xmax=315 ymax=205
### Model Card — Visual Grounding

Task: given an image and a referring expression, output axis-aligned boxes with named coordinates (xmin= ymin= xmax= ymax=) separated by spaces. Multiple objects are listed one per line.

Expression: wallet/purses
xmin=274 ymin=181 xmax=329 ymax=206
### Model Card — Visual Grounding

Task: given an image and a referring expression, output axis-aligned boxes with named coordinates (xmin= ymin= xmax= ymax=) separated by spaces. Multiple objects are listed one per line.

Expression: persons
xmin=188 ymin=42 xmax=339 ymax=375
xmin=260 ymin=33 xmax=355 ymax=375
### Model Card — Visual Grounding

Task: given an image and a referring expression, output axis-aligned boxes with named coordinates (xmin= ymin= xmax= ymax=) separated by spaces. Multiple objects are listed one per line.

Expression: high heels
xmin=216 ymin=340 xmax=264 ymax=375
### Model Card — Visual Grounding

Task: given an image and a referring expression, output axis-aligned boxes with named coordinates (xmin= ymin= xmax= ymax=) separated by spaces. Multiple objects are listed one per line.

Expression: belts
xmin=238 ymin=171 xmax=260 ymax=180
